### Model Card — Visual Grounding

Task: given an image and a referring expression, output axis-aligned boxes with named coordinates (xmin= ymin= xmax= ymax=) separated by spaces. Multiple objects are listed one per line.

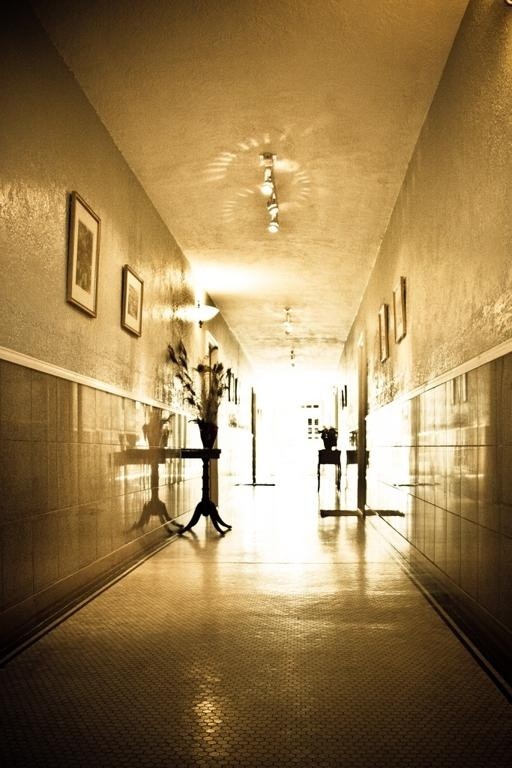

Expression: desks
xmin=177 ymin=450 xmax=232 ymax=536
xmin=124 ymin=448 xmax=183 ymax=534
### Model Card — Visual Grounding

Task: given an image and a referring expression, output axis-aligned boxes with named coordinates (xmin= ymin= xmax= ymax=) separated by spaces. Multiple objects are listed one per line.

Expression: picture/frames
xmin=121 ymin=265 xmax=144 ymax=336
xmin=67 ymin=190 xmax=101 ymax=319
xmin=378 ymin=276 xmax=406 ymax=367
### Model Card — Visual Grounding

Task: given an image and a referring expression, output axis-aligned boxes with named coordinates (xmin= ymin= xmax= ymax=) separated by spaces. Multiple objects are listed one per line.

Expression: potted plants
xmin=166 ymin=341 xmax=231 ymax=449
xmin=318 ymin=426 xmax=338 ymax=450
